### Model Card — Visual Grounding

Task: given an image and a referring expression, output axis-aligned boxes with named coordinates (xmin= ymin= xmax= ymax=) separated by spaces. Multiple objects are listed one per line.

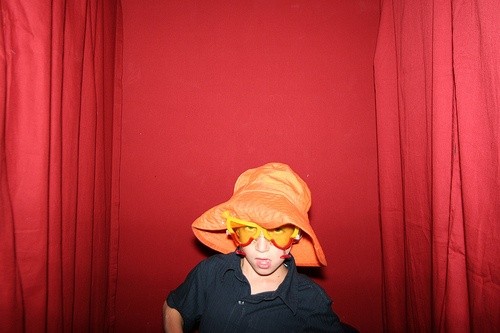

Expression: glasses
xmin=221 ymin=211 xmax=299 ymax=250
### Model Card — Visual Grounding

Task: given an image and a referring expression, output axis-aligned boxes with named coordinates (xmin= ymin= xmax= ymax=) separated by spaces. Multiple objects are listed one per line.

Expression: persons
xmin=161 ymin=162 xmax=359 ymax=333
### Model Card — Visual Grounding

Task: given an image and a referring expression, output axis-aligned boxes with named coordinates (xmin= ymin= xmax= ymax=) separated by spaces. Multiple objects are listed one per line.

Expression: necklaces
xmin=240 ymin=257 xmax=289 ymax=274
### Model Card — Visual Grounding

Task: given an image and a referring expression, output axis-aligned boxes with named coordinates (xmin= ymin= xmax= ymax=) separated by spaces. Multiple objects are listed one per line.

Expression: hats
xmin=191 ymin=162 xmax=328 ymax=267
